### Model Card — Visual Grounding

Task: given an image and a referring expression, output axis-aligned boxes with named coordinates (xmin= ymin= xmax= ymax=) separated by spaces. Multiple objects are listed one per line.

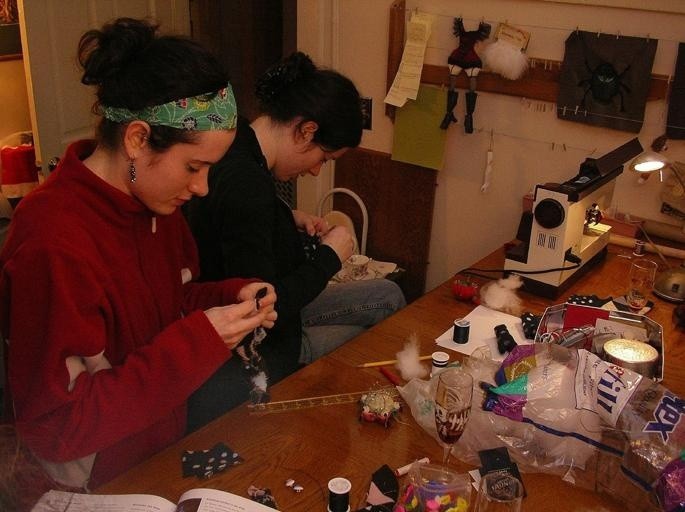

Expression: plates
xmin=338 ymin=269 xmax=376 ymax=281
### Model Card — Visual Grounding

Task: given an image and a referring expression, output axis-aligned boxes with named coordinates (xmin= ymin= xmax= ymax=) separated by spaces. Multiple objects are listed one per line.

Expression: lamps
xmin=629 ymin=151 xmax=685 ymax=195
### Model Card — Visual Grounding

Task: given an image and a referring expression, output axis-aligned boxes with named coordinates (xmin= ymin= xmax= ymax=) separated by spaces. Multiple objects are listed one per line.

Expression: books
xmin=25 ymin=488 xmax=280 ymax=512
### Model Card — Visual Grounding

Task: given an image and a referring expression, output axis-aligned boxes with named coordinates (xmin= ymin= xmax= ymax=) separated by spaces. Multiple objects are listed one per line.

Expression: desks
xmin=46 ymin=216 xmax=683 ymax=512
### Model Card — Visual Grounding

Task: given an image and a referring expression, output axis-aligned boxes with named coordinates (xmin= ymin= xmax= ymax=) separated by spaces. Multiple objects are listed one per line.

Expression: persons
xmin=2 ymin=16 xmax=279 ymax=493
xmin=175 ymin=50 xmax=412 ymax=438
xmin=439 ymin=15 xmax=491 ymax=134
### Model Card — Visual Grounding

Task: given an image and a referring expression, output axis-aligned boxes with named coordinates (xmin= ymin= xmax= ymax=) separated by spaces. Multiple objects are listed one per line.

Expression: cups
xmin=342 ymin=255 xmax=373 ymax=279
xmin=476 ymin=475 xmax=527 ymax=512
xmin=625 ymin=259 xmax=661 ymax=310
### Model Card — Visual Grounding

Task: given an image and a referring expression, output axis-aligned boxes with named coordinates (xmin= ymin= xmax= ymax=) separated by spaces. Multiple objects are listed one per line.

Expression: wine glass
xmin=429 ymin=369 xmax=475 ymax=477
